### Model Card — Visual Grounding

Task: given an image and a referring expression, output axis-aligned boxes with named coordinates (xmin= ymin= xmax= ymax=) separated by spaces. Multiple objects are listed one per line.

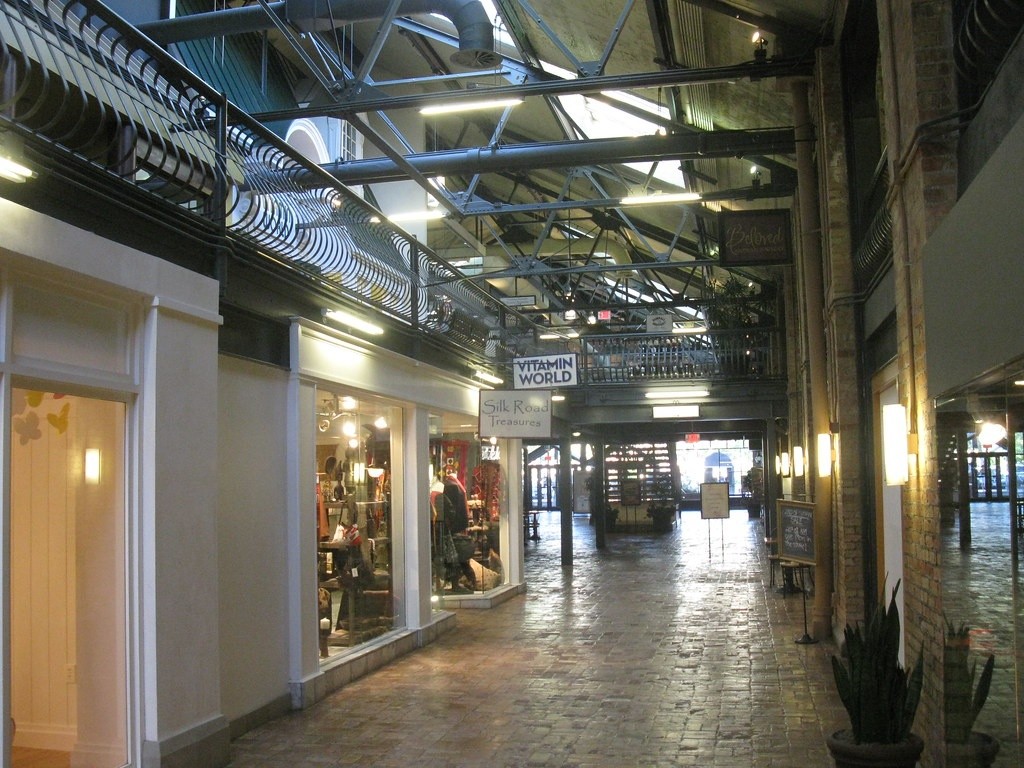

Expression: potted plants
xmin=743 ymin=464 xmax=765 ymax=519
xmin=824 ymin=572 xmax=924 ymax=768
xmin=646 ymin=473 xmax=683 ymax=532
xmin=584 ymin=471 xmax=619 ymax=533
xmin=943 ymin=611 xmax=1000 ymax=768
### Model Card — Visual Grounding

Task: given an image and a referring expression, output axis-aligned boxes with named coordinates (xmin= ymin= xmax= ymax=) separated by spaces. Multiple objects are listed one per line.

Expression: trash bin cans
xmin=748 ymin=500 xmax=761 ymax=521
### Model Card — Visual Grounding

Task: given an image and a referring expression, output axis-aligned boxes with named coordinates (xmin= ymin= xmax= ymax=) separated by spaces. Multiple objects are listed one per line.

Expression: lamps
xmin=966 ymin=393 xmax=1006 ymax=445
xmin=750 ymin=166 xmax=761 ymax=180
xmin=571 ymin=424 xmax=584 ymax=436
xmin=752 ymin=31 xmax=768 ymax=50
xmin=315 ymin=393 xmax=388 ymax=484
xmin=794 ymin=446 xmax=808 ymax=477
xmin=773 ymin=446 xmax=791 ymax=478
xmin=749 ymin=282 xmax=756 ymax=292
xmin=881 ymin=403 xmax=919 ymax=487
xmin=85 ymin=448 xmax=103 ymax=486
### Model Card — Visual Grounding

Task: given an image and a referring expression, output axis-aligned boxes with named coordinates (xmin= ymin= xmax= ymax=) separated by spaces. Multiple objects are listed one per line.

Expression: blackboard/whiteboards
xmin=777 ymin=499 xmax=819 ymax=564
xmin=478 ymin=388 xmax=552 ymax=440
xmin=700 ymin=482 xmax=730 ymax=519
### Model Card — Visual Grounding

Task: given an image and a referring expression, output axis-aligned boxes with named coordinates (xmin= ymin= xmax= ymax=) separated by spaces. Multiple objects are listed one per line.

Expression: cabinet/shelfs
xmin=318 ymin=499 xmax=393 ymax=647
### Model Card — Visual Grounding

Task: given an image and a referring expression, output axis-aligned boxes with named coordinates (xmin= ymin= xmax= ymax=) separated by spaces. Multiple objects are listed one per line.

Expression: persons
xmin=430 ymin=469 xmax=476 ymax=595
